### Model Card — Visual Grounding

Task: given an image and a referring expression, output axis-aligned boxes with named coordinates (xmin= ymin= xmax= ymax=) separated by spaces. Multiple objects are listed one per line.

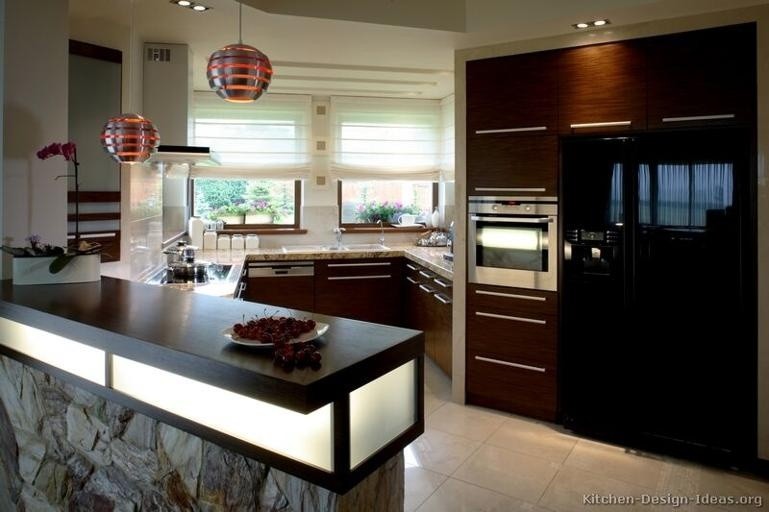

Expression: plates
xmin=390 ymin=224 xmax=423 ymax=228
xmin=221 ymin=321 xmax=329 ymax=347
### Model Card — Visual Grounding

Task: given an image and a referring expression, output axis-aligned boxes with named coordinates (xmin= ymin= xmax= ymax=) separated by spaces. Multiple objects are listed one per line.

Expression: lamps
xmin=207 ymin=0 xmax=273 ymax=104
xmin=100 ymin=0 xmax=161 ymax=165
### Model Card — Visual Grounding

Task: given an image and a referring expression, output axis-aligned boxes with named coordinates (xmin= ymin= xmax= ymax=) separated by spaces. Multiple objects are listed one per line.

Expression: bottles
xmin=432 ymin=206 xmax=441 ymax=228
xmin=188 ymin=215 xmax=260 ymax=251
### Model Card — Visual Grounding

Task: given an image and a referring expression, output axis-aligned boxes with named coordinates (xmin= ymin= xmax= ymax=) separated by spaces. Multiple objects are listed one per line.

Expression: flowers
xmin=213 ymin=198 xmax=280 ymax=220
xmin=353 ymin=200 xmax=412 ymax=223
xmin=0 ymin=141 xmax=100 ymax=274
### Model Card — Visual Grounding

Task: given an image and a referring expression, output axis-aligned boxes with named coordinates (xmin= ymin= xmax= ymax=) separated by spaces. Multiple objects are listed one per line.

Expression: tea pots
xmin=398 ymin=213 xmax=418 ymax=225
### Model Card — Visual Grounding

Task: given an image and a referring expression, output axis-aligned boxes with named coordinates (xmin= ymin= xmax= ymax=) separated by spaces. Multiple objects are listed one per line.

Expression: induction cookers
xmin=146 ymin=263 xmax=235 ymax=289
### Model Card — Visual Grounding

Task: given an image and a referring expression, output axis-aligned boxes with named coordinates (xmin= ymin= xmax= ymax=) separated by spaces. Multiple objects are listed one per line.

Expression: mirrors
xmin=67 ymin=38 xmax=122 ymax=263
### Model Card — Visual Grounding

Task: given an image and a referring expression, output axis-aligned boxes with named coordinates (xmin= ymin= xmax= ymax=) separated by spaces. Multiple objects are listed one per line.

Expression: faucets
xmin=376 ymin=218 xmax=384 ymax=238
xmin=334 ymin=226 xmax=343 ymax=243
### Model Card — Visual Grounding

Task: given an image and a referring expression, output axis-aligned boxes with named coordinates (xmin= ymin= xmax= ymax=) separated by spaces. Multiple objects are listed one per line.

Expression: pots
xmin=164 ymin=262 xmax=206 ymax=279
xmin=165 ymin=241 xmax=196 ymax=265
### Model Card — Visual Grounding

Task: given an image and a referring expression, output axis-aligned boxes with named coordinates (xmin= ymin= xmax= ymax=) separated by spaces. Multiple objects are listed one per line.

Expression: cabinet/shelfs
xmin=236 ymin=260 xmax=248 ymax=301
xmin=248 ymin=260 xmax=314 ymax=313
xmin=314 ymin=257 xmax=403 ymax=327
xmin=403 ymin=256 xmax=453 ymax=382
xmin=559 ymin=37 xmax=647 ymax=134
xmin=466 ymin=49 xmax=559 ymax=196
xmin=647 ymin=21 xmax=757 ymax=129
xmin=465 ymin=283 xmax=558 ymax=424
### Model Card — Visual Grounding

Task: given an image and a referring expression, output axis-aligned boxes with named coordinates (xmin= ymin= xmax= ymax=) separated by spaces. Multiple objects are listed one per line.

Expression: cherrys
xmin=233 ymin=309 xmax=322 ymax=368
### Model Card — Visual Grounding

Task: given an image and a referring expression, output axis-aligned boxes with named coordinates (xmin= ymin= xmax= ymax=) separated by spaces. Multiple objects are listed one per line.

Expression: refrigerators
xmin=558 ymin=129 xmax=755 ymax=463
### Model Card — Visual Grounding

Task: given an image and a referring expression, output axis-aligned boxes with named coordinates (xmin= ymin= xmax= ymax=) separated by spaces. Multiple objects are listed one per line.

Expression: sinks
xmin=343 ymin=243 xmax=391 ymax=251
xmin=325 ymin=245 xmax=343 ymax=252
xmin=280 ymin=243 xmax=329 ymax=254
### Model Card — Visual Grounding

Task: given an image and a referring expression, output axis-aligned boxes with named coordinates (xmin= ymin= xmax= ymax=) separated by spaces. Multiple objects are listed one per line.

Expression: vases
xmin=369 ymin=214 xmax=388 ymax=222
xmin=2 ymin=250 xmax=101 ymax=286
xmin=217 ymin=216 xmax=274 ymax=224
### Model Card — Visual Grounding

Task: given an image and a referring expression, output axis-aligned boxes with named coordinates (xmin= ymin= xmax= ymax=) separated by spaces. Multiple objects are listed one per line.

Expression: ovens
xmin=467 ymin=196 xmax=559 ymax=296
